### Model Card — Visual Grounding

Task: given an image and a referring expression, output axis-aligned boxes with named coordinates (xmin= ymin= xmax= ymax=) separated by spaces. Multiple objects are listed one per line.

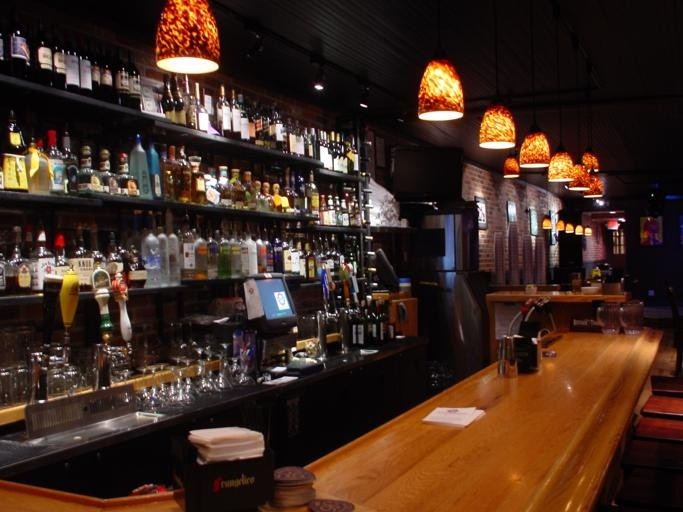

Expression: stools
xmin=615 ymin=377 xmax=683 ymax=512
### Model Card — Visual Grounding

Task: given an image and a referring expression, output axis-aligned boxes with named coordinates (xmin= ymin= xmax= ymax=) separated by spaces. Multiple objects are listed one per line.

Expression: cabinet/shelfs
xmin=0 ymin=65 xmax=369 ymax=429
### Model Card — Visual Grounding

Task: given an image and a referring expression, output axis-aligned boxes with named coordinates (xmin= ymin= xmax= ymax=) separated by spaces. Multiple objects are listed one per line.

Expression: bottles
xmin=0 ymin=20 xmax=395 ymax=419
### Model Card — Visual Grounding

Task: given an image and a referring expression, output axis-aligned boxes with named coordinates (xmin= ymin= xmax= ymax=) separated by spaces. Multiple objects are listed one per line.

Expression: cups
xmin=514 ymin=319 xmax=540 ymax=374
xmin=596 ymin=303 xmax=622 ymax=335
xmin=618 ymin=302 xmax=644 ymax=334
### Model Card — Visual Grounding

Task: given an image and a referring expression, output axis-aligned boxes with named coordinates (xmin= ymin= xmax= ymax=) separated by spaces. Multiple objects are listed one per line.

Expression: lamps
xmin=519 ymin=0 xmax=551 ymax=168
xmin=503 ymin=151 xmax=521 ymax=178
xmin=542 ymin=215 xmax=592 ymax=237
xmin=548 ymin=16 xmax=609 ymax=198
xmin=478 ymin=0 xmax=517 ymax=149
xmin=155 ymin=0 xmax=221 ymax=75
xmin=416 ymin=0 xmax=465 ymax=121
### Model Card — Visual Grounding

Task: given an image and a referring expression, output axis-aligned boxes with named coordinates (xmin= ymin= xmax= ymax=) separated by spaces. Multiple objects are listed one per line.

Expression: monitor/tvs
xmin=244 ymin=271 xmax=298 ymax=340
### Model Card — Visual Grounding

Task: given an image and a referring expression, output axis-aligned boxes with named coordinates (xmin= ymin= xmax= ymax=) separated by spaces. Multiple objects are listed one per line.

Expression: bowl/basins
xmin=524 ymin=288 xmax=537 ymax=295
xmin=580 ymin=287 xmax=598 ymax=294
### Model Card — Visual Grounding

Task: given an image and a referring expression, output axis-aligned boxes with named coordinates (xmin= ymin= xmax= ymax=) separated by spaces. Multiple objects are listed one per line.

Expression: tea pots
xmin=497 ymin=336 xmax=517 ymax=378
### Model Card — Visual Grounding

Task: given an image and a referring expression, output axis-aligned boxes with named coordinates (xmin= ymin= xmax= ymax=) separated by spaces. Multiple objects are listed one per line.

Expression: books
xmin=422 ymin=406 xmax=486 ymax=428
